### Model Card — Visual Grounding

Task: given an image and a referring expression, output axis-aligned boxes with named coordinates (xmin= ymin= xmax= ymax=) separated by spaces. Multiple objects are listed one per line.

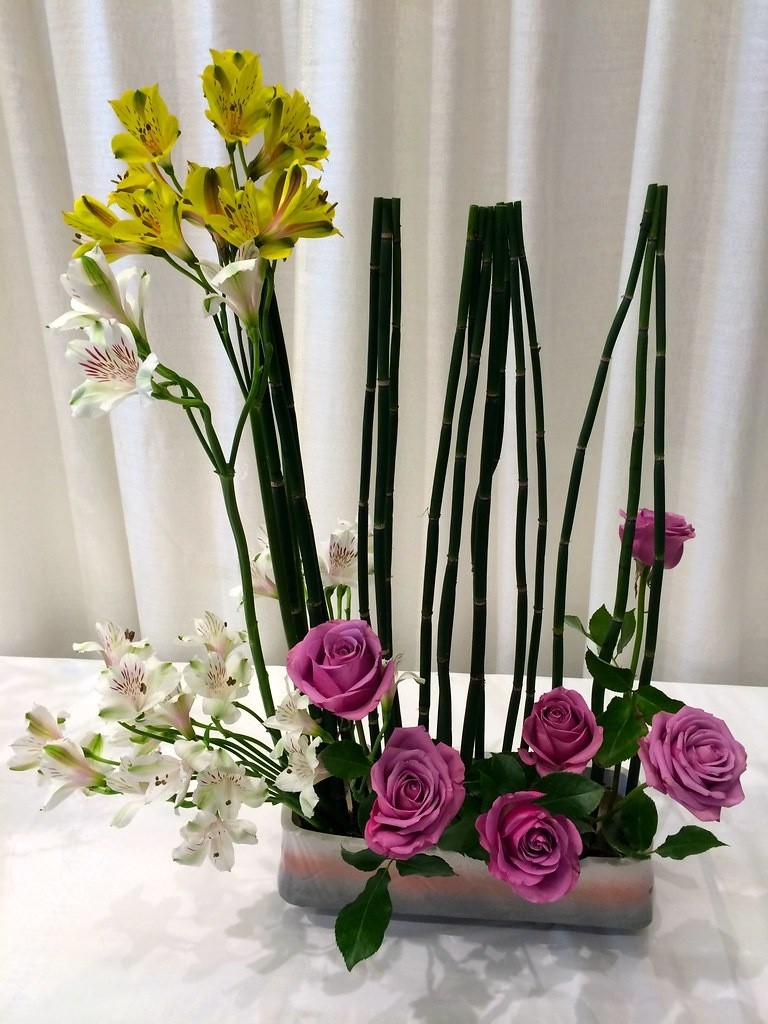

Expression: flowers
xmin=6 ymin=47 xmax=747 ymax=972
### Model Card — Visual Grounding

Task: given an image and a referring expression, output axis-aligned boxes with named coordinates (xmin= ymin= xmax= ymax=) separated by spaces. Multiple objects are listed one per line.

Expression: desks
xmin=1 ymin=655 xmax=768 ymax=1024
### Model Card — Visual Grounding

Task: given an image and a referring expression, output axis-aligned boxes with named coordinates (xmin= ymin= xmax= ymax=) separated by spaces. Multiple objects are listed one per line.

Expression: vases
xmin=276 ymin=751 xmax=654 ymax=931
